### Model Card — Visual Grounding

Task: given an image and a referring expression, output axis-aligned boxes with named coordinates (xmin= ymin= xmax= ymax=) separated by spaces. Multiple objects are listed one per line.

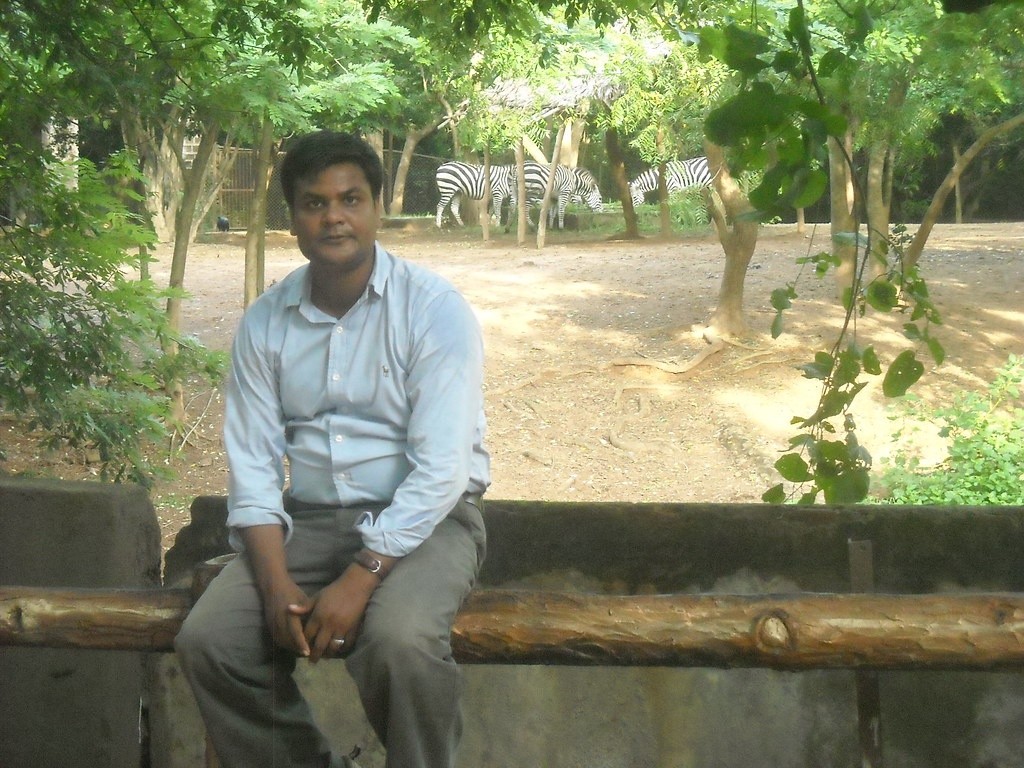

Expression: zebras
xmin=630 ymin=156 xmax=714 ymax=208
xmin=504 ymin=161 xmax=603 ymax=234
xmin=435 ymin=161 xmax=515 ymax=230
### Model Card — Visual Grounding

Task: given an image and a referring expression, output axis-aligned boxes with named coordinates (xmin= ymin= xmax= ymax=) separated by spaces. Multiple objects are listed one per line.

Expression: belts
xmin=462 ymin=491 xmax=481 ymax=511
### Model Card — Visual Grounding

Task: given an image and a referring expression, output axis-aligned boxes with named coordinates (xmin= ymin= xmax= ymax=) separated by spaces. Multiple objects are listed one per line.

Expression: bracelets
xmin=354 ymin=550 xmax=389 ymax=580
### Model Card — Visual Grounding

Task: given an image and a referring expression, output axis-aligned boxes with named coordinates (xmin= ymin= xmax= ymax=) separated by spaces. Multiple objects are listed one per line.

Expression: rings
xmin=331 ymin=638 xmax=345 ymax=648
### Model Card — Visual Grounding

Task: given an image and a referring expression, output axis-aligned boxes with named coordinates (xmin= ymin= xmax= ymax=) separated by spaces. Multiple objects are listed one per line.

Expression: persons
xmin=176 ymin=130 xmax=490 ymax=767
